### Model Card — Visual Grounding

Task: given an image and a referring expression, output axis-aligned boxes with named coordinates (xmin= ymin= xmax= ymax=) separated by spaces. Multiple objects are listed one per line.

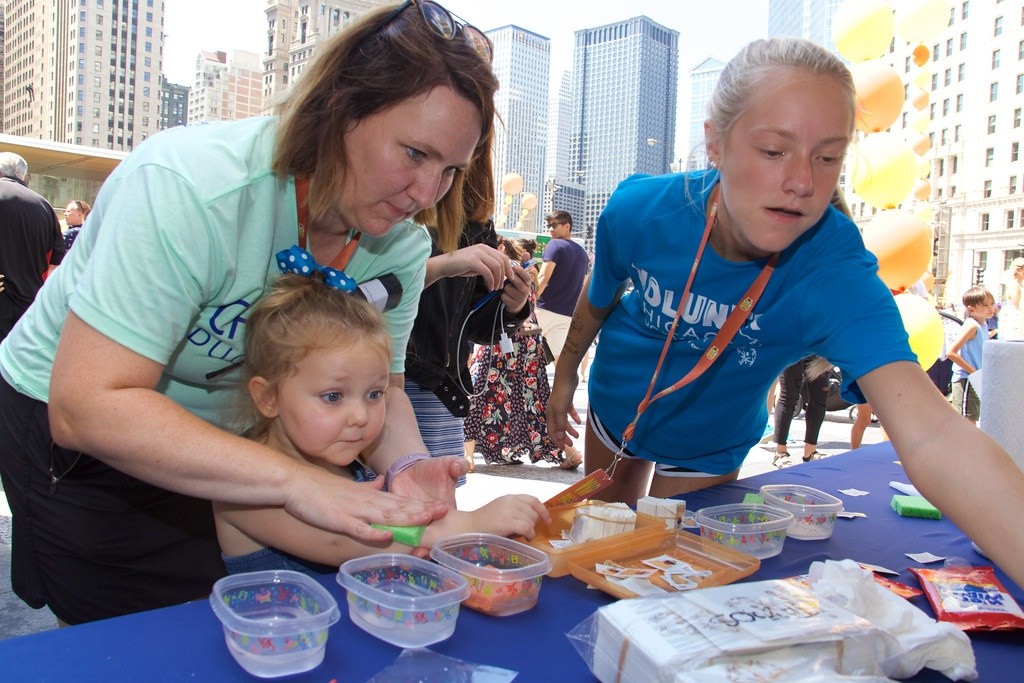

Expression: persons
xmin=761 ymin=256 xmax=1024 ymax=469
xmin=212 ymin=272 xmax=551 ymax=577
xmin=0 ymin=150 xmax=592 ymax=471
xmin=0 ymin=0 xmax=500 ymax=629
xmin=544 ymin=38 xmax=1024 ymax=594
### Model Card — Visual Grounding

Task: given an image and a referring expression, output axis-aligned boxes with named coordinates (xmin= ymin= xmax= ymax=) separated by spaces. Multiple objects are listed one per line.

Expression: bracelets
xmin=384 ymin=453 xmax=431 ymax=493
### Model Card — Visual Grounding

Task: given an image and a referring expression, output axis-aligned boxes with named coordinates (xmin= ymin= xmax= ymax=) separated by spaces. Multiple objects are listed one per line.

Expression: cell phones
xmin=471 ymin=259 xmax=537 ymax=313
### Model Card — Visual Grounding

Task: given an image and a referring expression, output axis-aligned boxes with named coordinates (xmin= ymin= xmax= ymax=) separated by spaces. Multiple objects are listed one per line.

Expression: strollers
xmin=774 ymin=365 xmax=880 ymax=425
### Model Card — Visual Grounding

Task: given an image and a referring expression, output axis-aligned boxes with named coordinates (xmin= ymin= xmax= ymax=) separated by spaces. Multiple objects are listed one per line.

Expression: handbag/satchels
xmin=461 ymin=263 xmax=563 ymax=463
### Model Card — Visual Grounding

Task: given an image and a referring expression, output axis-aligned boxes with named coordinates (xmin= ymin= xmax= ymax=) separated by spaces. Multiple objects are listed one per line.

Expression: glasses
xmin=547 ymin=223 xmax=563 ymax=229
xmin=359 ymin=0 xmax=494 ymax=66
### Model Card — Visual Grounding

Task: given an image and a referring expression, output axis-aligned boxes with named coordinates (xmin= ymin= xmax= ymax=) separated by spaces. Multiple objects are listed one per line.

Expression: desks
xmin=0 ymin=442 xmax=1024 ymax=683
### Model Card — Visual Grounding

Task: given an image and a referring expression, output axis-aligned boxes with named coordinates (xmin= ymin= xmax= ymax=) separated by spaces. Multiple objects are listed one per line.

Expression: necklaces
xmin=296 ymin=175 xmax=361 ymax=271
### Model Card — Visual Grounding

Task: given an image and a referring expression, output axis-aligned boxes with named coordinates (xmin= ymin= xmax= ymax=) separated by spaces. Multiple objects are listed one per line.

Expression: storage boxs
xmin=336 ymin=553 xmax=469 ymax=649
xmin=758 ymin=483 xmax=843 ymax=540
xmin=432 ymin=529 xmax=551 ymax=617
xmin=690 ymin=502 xmax=793 ymax=559
xmin=209 ymin=569 xmax=338 ymax=679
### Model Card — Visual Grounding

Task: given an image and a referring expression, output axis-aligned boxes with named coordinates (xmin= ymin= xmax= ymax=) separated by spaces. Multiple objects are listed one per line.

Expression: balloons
xmin=494 ymin=172 xmax=523 ymax=228
xmin=516 ymin=192 xmax=537 ymax=231
xmin=829 ymin=0 xmax=947 ymax=373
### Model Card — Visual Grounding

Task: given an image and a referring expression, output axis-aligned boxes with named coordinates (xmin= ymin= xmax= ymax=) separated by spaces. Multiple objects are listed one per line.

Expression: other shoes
xmin=771 ymin=451 xmax=793 ymax=469
xmin=802 ymin=451 xmax=828 ymax=463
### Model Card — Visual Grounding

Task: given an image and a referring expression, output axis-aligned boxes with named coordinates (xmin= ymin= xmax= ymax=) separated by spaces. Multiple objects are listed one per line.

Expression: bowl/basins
xmin=429 ymin=532 xmax=553 ymax=617
xmin=210 ymin=570 xmax=341 ymax=679
xmin=759 ymin=483 xmax=844 ymax=541
xmin=696 ymin=503 xmax=794 ymax=560
xmin=509 ymin=499 xmax=666 ymax=577
xmin=336 ymin=554 xmax=470 ymax=648
